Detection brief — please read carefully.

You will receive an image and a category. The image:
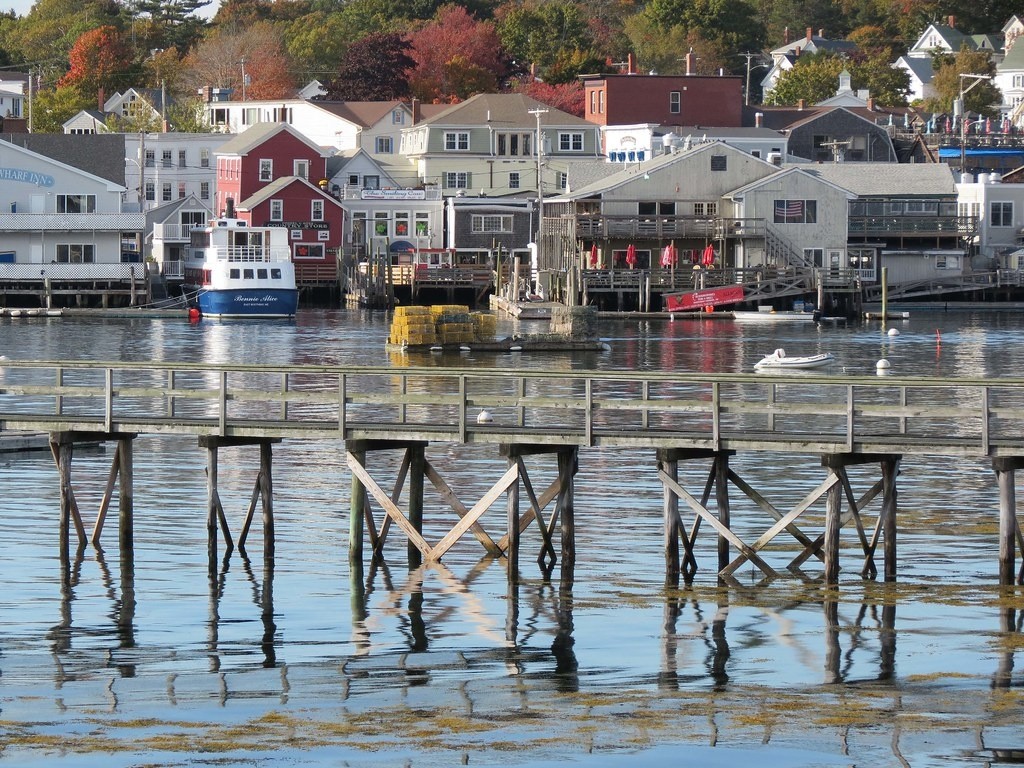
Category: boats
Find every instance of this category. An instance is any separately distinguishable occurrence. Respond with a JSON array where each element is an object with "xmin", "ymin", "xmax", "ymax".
[
  {"xmin": 385, "ymin": 333, "xmax": 603, "ymax": 352},
  {"xmin": 732, "ymin": 311, "xmax": 814, "ymax": 320},
  {"xmin": 181, "ymin": 198, "xmax": 300, "ymax": 318},
  {"xmin": 754, "ymin": 349, "xmax": 835, "ymax": 368}
]
